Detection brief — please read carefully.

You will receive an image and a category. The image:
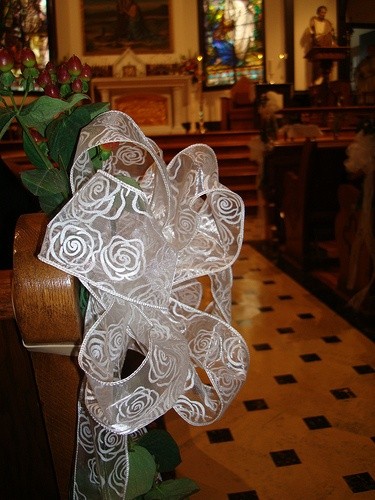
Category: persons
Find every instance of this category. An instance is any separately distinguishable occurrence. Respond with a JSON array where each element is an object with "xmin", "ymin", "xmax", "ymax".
[{"xmin": 309, "ymin": 5, "xmax": 334, "ymax": 85}]
